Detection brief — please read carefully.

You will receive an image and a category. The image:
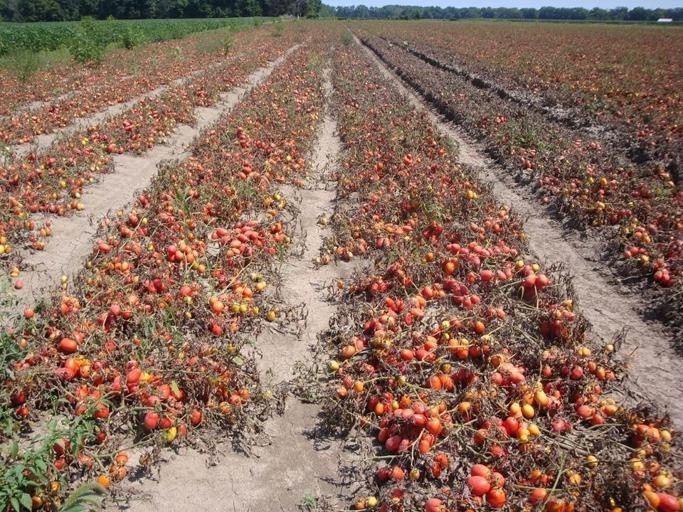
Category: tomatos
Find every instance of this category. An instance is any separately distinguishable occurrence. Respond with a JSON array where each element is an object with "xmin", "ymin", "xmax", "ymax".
[{"xmin": 0, "ymin": 88, "xmax": 683, "ymax": 512}]
